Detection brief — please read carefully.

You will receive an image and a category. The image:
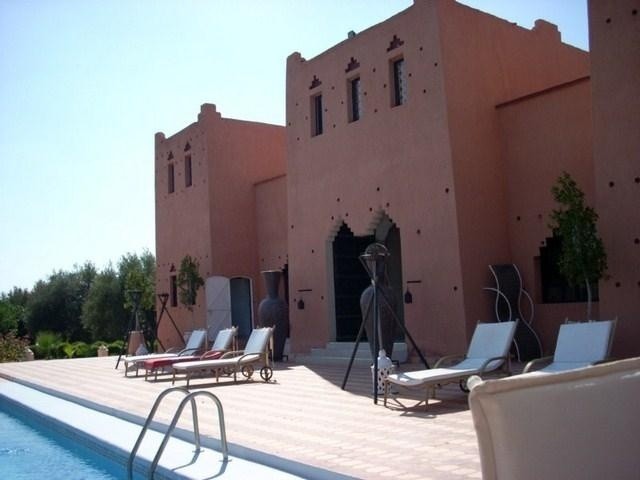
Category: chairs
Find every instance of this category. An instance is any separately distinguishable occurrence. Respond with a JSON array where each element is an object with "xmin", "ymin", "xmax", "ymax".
[
  {"xmin": 523, "ymin": 316, "xmax": 619, "ymax": 374},
  {"xmin": 468, "ymin": 356, "xmax": 640, "ymax": 480},
  {"xmin": 122, "ymin": 325, "xmax": 278, "ymax": 388},
  {"xmin": 384, "ymin": 319, "xmax": 518, "ymax": 412},
  {"xmin": 484, "ymin": 263, "xmax": 544, "ymax": 363}
]
